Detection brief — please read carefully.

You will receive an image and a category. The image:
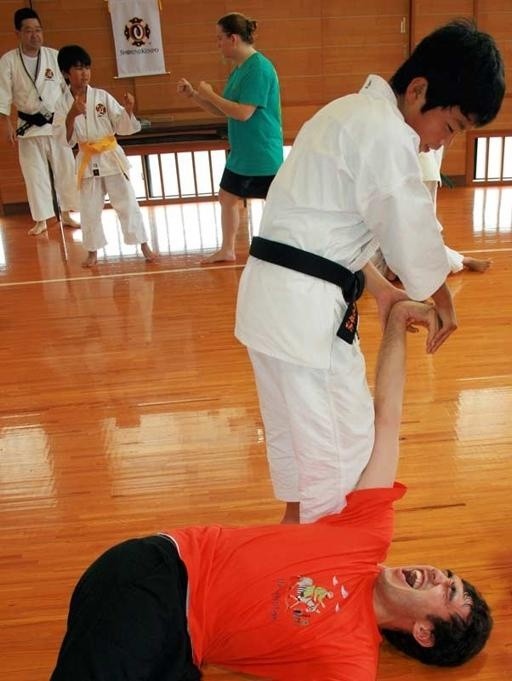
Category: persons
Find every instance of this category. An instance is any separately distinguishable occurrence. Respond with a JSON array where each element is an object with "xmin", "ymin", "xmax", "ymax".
[
  {"xmin": 45, "ymin": 299, "xmax": 493, "ymax": 681},
  {"xmin": 233, "ymin": 15, "xmax": 506, "ymax": 526},
  {"xmin": 176, "ymin": 11, "xmax": 283, "ymax": 265},
  {"xmin": 0, "ymin": 8, "xmax": 82, "ymax": 236},
  {"xmin": 359, "ymin": 142, "xmax": 493, "ymax": 282},
  {"xmin": 52, "ymin": 45, "xmax": 154, "ymax": 268}
]
